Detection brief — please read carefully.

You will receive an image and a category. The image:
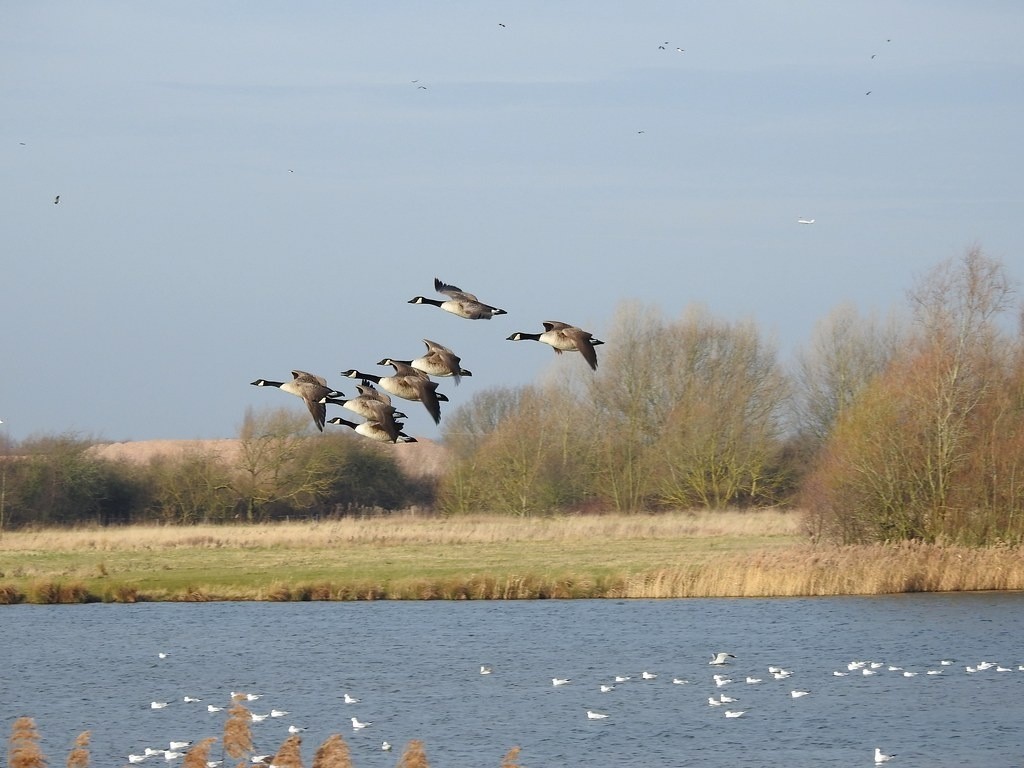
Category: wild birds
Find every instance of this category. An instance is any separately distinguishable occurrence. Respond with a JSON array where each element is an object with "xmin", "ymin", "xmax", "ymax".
[
  {"xmin": 586, "ymin": 651, "xmax": 1023, "ymax": 721},
  {"xmin": 127, "ymin": 651, "xmax": 392, "ymax": 768},
  {"xmin": 551, "ymin": 677, "xmax": 572, "ymax": 686},
  {"xmin": 874, "ymin": 748, "xmax": 898, "ymax": 763},
  {"xmin": 505, "ymin": 320, "xmax": 606, "ymax": 372},
  {"xmin": 249, "ymin": 337, "xmax": 474, "ymax": 445},
  {"xmin": 479, "ymin": 665, "xmax": 494, "ymax": 675},
  {"xmin": 407, "ymin": 278, "xmax": 507, "ymax": 321}
]
